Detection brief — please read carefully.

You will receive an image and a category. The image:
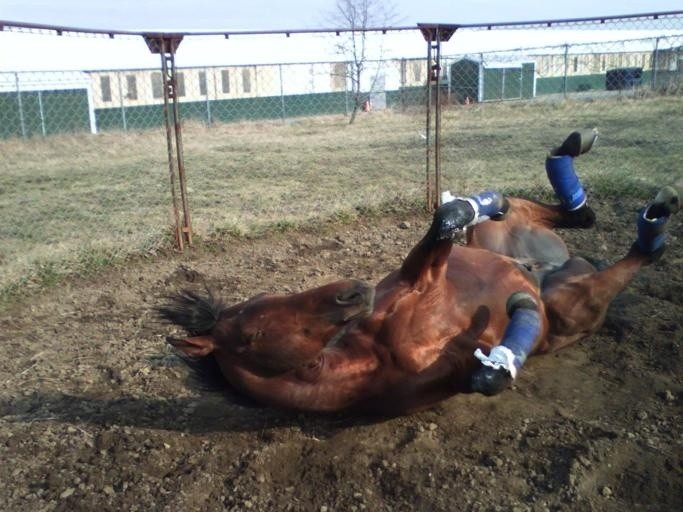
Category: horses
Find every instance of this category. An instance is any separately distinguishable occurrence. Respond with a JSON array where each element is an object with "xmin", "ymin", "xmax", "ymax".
[{"xmin": 149, "ymin": 126, "xmax": 683, "ymax": 419}]
